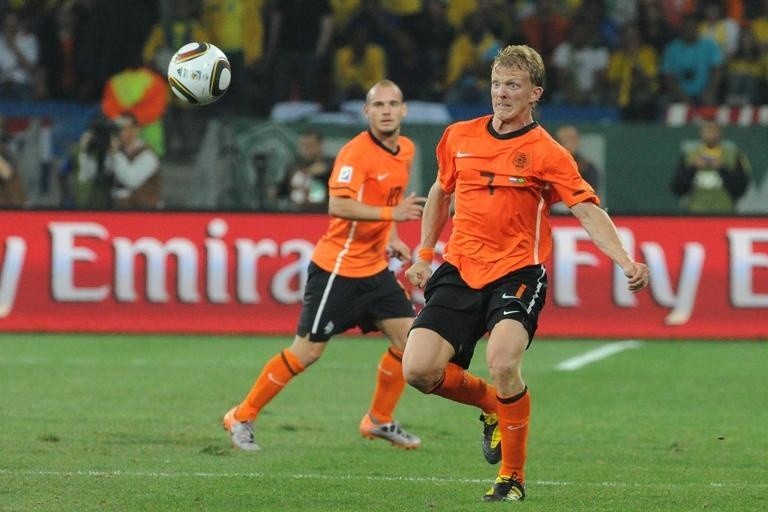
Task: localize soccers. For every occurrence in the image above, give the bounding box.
[167,42,233,105]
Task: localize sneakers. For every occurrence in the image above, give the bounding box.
[358,413,420,450]
[480,477,525,503]
[479,410,504,466]
[221,405,261,452]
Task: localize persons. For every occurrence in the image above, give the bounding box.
[0,1,767,211]
[222,77,428,452]
[399,43,649,499]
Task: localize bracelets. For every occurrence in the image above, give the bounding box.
[418,248,435,265]
